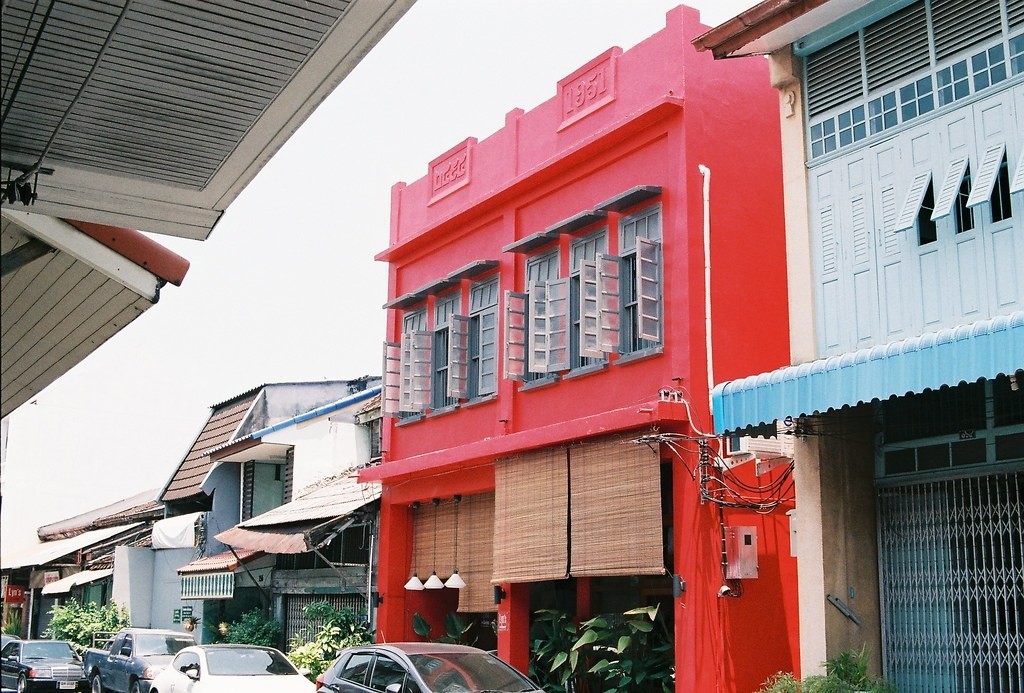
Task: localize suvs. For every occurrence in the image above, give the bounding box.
[315,642,546,693]
[148,643,317,693]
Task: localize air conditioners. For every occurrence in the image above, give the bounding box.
[726,417,796,458]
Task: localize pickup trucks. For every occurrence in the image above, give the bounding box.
[83,627,197,693]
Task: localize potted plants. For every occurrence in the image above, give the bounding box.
[185,616,201,631]
[219,622,228,635]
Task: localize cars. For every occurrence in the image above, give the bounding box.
[0,639,92,693]
[0,634,22,650]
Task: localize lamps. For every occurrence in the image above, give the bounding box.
[404,501,425,590]
[444,494,467,588]
[423,497,444,588]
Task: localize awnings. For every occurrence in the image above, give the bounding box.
[177,509,371,605]
[712,310,1024,437]
[41,570,113,595]
